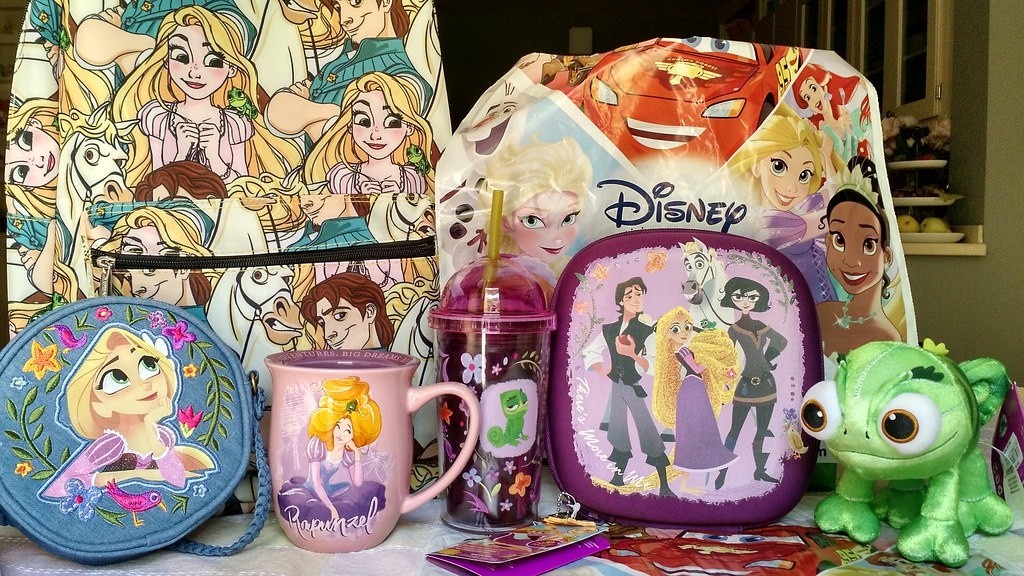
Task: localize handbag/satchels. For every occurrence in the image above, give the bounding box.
[0,296,274,565]
[443,40,919,493]
[545,228,825,525]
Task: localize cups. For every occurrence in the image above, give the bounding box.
[428,256,557,533]
[265,349,483,554]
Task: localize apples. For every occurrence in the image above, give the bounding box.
[898,215,950,233]
